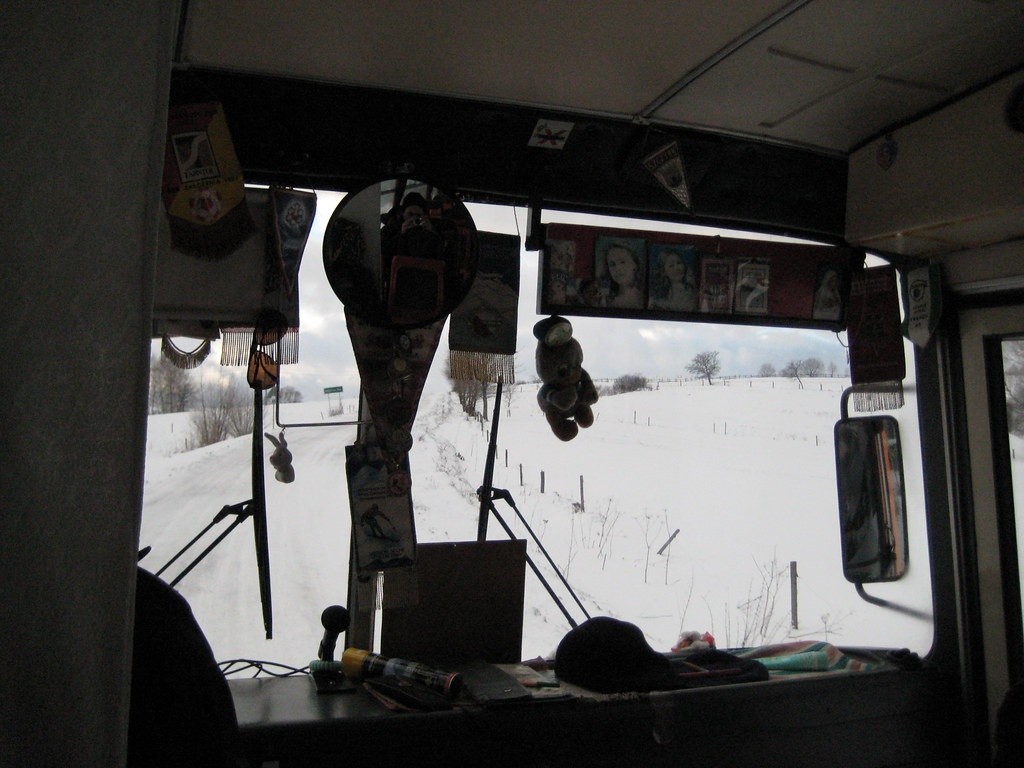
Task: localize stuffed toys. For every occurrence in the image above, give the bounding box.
[532,315,599,442]
[264,425,296,483]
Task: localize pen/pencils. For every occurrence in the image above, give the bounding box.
[520,680,560,689]
[309,660,341,671]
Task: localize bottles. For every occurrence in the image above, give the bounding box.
[340,648,461,694]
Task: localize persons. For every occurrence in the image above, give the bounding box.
[133,546,248,768]
[359,504,393,538]
[383,192,445,260]
[602,240,701,312]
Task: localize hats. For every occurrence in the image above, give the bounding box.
[553,617,676,694]
[402,192,426,212]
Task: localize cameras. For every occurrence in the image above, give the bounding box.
[413,216,427,226]
